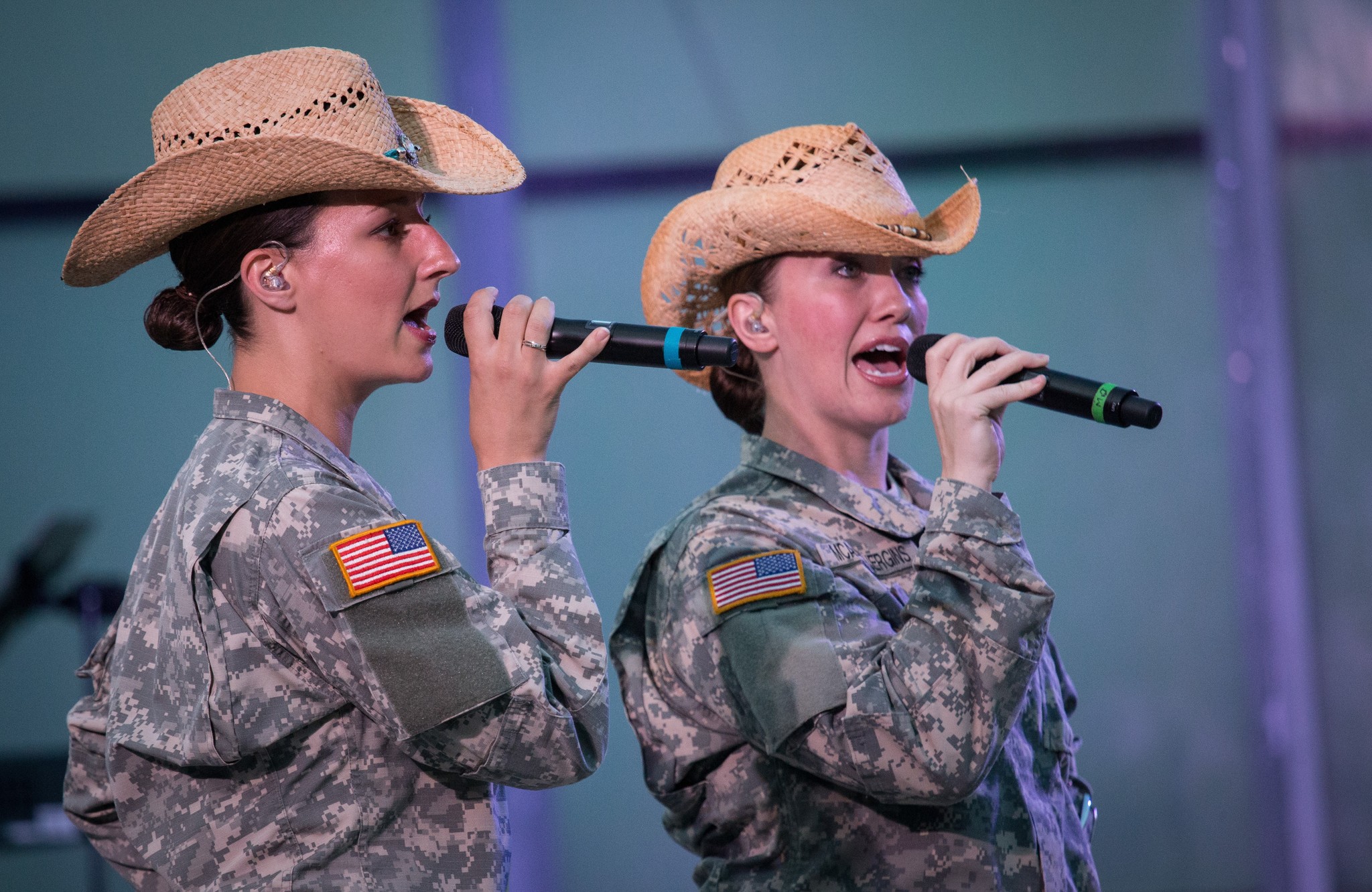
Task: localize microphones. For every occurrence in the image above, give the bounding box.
[442,304,741,370]
[907,333,1164,430]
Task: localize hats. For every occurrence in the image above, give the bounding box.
[639,122,985,394]
[62,53,523,288]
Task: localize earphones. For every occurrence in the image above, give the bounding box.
[261,264,285,290]
[746,309,767,333]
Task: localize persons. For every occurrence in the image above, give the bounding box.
[61,41,611,890]
[608,119,1105,889]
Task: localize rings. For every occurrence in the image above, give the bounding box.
[522,340,546,353]
